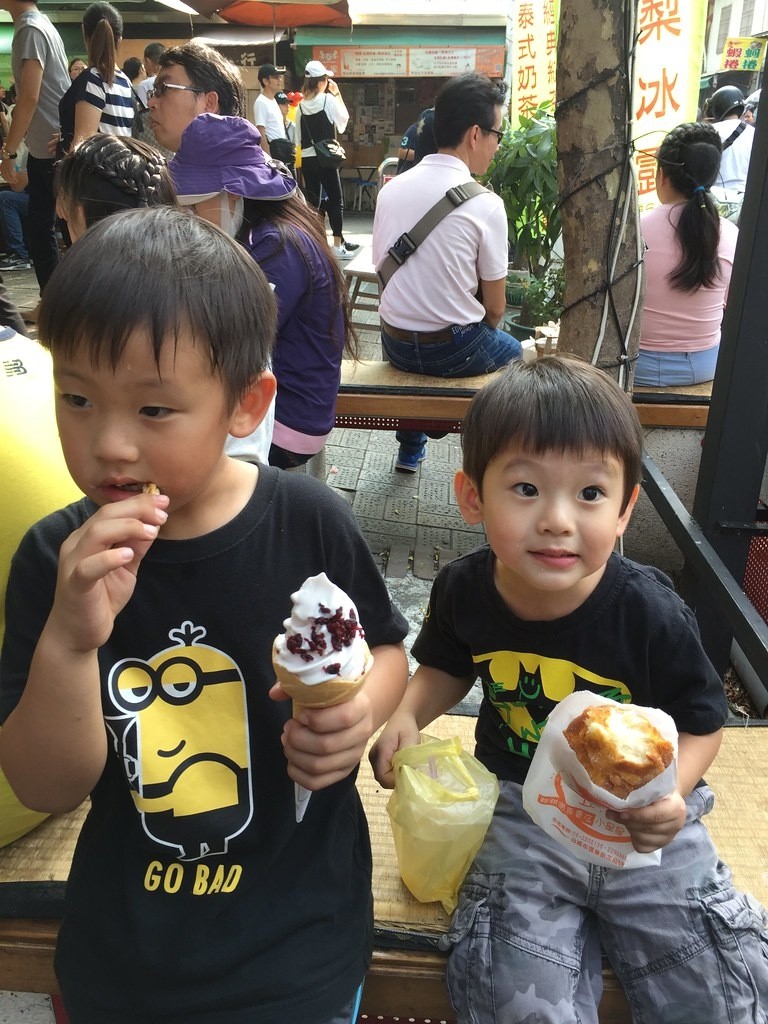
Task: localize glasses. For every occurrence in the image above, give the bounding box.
[472,122,504,145]
[264,76,281,81]
[145,84,210,100]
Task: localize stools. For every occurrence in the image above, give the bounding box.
[341,178,377,212]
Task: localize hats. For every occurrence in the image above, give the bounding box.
[258,65,287,81]
[305,60,334,79]
[165,112,297,205]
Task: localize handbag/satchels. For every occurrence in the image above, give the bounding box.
[314,138,346,170]
[386,733,500,916]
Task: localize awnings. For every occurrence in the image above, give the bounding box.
[292,24,507,80]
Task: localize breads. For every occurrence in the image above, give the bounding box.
[563,703,673,801]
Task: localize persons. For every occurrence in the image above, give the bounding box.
[56,135,177,241]
[373,73,523,472]
[0,208,410,1024]
[370,358,768,1024]
[123,57,147,86]
[633,123,738,386]
[137,43,166,109]
[58,2,135,169]
[147,44,247,151]
[297,60,363,259]
[696,86,761,191]
[274,92,296,148]
[167,113,357,469]
[253,64,295,180]
[68,58,88,80]
[0,0,73,337]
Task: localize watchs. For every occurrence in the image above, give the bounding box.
[0,150,18,160]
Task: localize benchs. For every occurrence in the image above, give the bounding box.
[284,360,712,572]
[342,246,382,331]
[0,728,768,1024]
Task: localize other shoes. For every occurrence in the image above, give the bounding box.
[344,242,358,251]
[18,299,43,322]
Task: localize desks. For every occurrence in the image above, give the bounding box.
[342,166,378,210]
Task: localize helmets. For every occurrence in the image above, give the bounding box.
[710,85,744,121]
[706,98,713,118]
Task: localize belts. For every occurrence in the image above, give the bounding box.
[379,317,455,345]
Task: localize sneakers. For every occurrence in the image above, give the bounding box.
[394,448,426,474]
[333,245,355,260]
[0,253,31,271]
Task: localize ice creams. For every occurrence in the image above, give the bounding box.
[271,572,374,721]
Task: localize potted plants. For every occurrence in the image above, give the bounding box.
[471,99,567,342]
